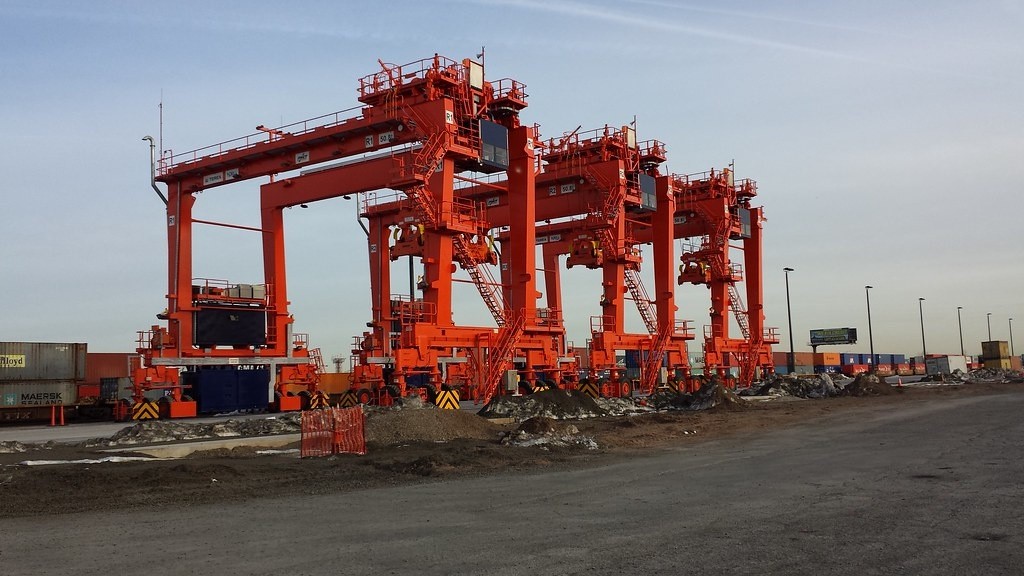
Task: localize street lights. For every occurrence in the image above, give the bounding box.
[957,306,965,356]
[864,285,876,371]
[919,297,929,376]
[783,266,796,372]
[1008,318,1014,356]
[987,313,993,341]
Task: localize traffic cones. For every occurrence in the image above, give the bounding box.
[46,404,58,427]
[57,404,69,427]
[897,378,902,387]
